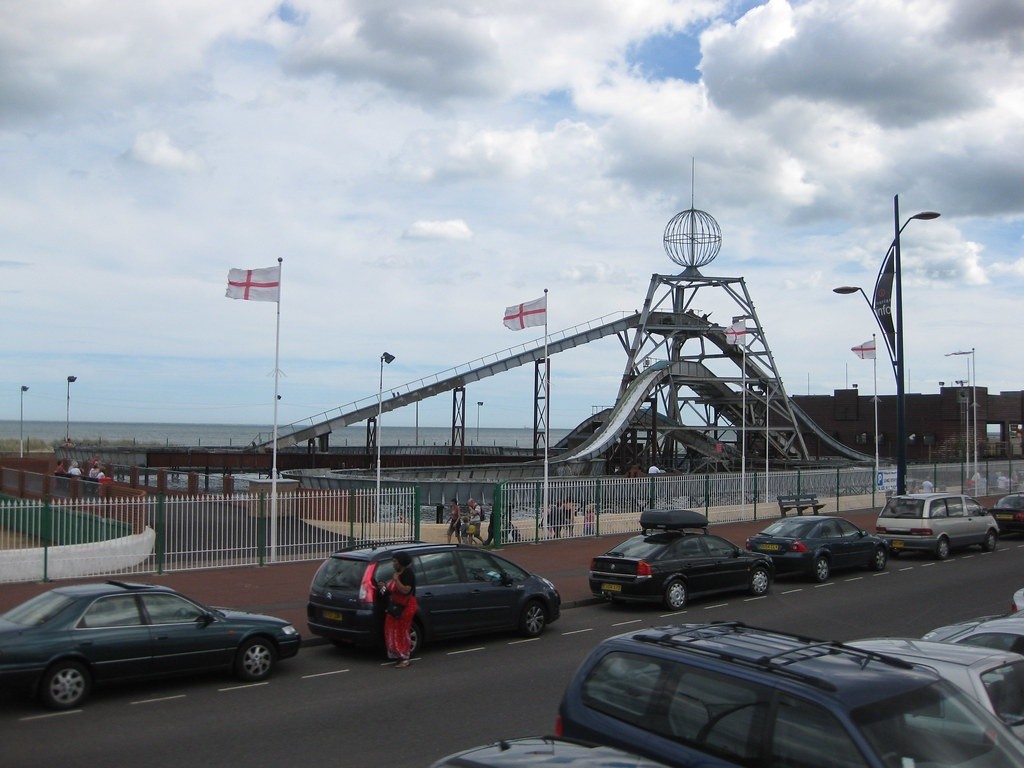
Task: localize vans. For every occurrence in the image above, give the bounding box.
[876,492,1000,561]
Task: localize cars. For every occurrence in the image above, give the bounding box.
[986,494,1024,532]
[427,734,669,768]
[919,612,1024,659]
[842,636,1024,747]
[1011,586,1024,613]
[586,510,776,610]
[0,579,301,712]
[745,515,890,583]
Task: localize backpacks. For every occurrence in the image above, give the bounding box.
[471,505,485,521]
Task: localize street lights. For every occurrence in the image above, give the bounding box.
[477,401,483,441]
[375,351,397,525]
[833,193,942,496]
[19,386,30,458]
[66,376,78,442]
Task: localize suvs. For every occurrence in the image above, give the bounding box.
[307,540,562,656]
[555,621,1024,768]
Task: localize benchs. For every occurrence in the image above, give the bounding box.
[777,493,824,518]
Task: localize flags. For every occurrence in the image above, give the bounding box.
[723,321,745,345]
[224,266,281,302]
[851,340,876,359]
[504,294,547,331]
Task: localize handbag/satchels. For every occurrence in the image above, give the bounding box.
[388,601,405,619]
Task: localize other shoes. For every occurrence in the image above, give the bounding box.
[395,660,410,668]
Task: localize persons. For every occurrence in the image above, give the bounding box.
[57,456,105,478]
[884,469,1018,502]
[446,498,521,545]
[377,551,417,667]
[540,498,595,539]
